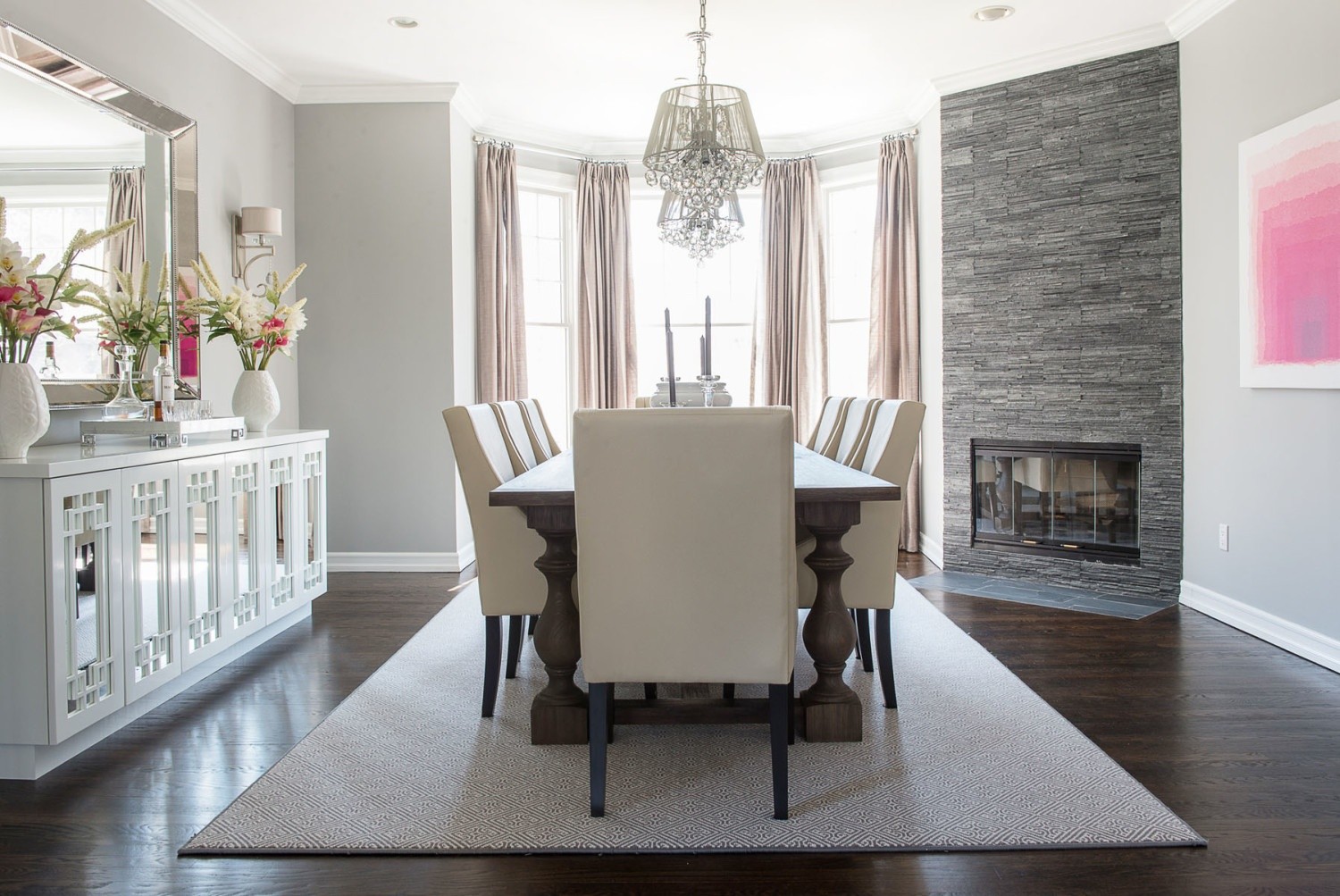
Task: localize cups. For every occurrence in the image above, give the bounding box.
[161,400,210,421]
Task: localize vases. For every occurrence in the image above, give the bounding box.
[232,370,280,432]
[0,363,50,459]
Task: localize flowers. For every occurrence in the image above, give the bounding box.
[66,250,171,372]
[179,253,308,370]
[0,201,138,363]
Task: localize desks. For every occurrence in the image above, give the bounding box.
[489,443,901,743]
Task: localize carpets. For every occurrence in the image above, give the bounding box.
[178,573,1210,857]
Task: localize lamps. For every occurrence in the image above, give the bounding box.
[643,0,765,266]
[231,206,282,296]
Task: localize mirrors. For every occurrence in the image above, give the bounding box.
[0,59,177,379]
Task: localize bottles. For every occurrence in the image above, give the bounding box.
[102,345,149,421]
[41,341,60,379]
[152,340,176,421]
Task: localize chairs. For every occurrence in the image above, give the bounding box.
[444,396,926,821]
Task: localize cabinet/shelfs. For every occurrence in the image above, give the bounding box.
[0,430,329,779]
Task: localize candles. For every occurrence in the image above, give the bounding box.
[664,307,676,407]
[700,295,711,375]
